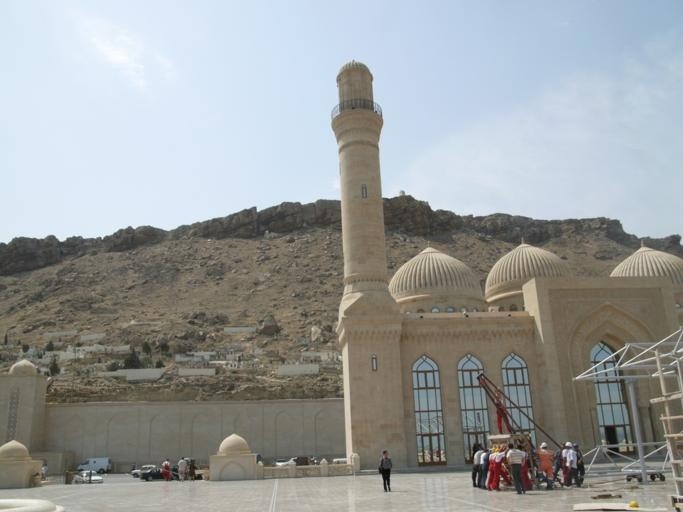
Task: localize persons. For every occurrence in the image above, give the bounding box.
[40,463,45,481]
[161,456,170,481]
[177,456,187,480]
[377,449,392,493]
[189,458,199,480]
[470,440,585,493]
[42,463,48,475]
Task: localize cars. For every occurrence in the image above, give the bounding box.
[130,464,155,477]
[74,470,102,482]
[138,466,178,481]
[271,458,287,467]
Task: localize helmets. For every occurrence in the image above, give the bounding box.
[564,442,572,447]
[541,442,548,447]
[573,443,579,449]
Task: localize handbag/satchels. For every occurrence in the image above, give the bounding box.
[378,466,384,474]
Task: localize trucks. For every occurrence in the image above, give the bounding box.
[76,457,111,473]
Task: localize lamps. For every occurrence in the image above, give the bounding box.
[371,353,377,372]
